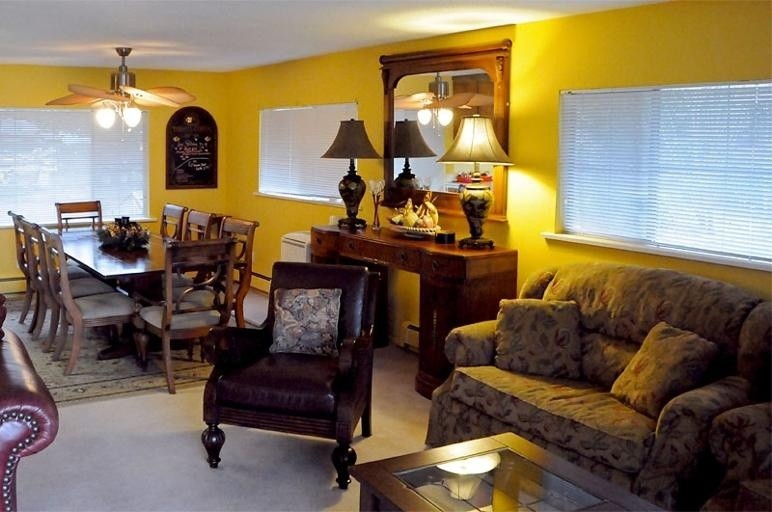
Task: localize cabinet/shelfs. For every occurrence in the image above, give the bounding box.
[310,229,517,400]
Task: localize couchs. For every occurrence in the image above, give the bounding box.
[0,293,58,512]
[425,263,772,510]
[699,402,772,511]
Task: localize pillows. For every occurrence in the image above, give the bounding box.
[269,287,343,358]
[611,321,718,421]
[494,297,583,382]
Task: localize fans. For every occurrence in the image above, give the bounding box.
[44,47,197,110]
[394,72,494,109]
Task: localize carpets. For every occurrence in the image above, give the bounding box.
[3,311,265,407]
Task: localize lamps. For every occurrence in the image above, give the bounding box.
[435,451,501,502]
[432,114,515,253]
[320,118,382,230]
[392,118,436,189]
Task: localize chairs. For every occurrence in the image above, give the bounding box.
[39,228,142,376]
[18,216,118,352]
[7,211,65,333]
[134,215,259,359]
[160,202,190,241]
[202,261,382,489]
[55,200,103,234]
[138,237,237,395]
[139,209,216,298]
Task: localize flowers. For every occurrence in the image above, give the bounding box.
[97,219,149,255]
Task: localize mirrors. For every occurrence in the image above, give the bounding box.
[379,39,512,223]
